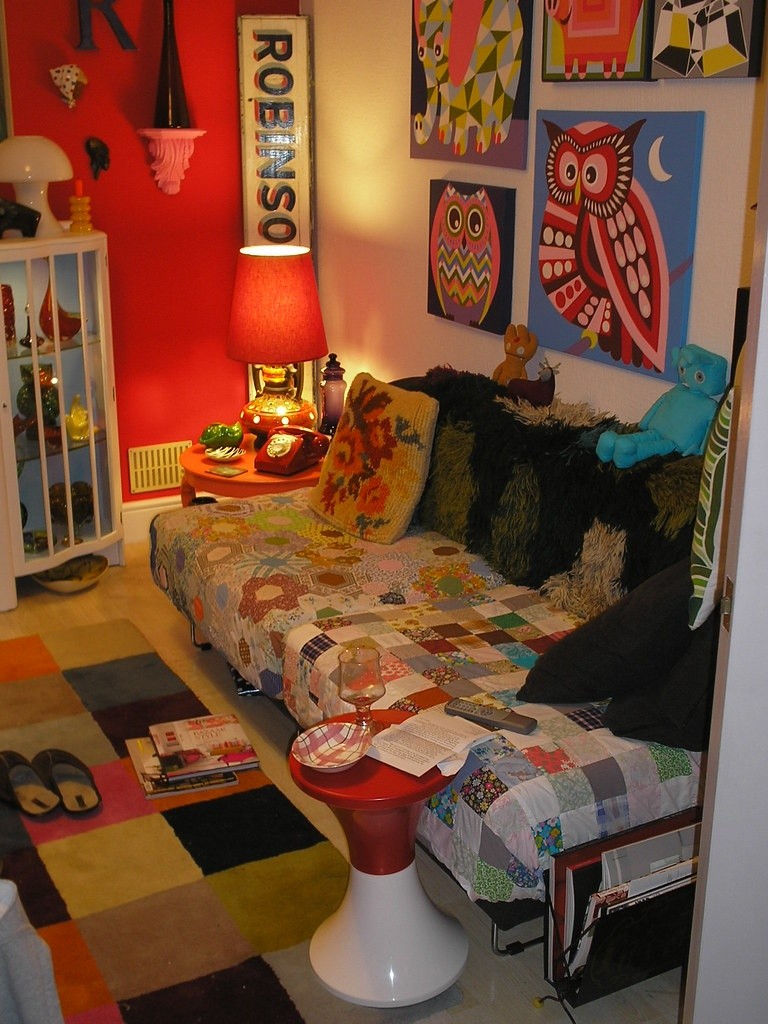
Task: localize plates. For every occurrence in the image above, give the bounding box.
[205,447,246,461]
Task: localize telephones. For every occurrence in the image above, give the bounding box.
[254,424,329,476]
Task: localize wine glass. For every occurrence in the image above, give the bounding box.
[338,647,386,736]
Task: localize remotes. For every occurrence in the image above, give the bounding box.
[444,697,537,735]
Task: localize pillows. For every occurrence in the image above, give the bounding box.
[310,372,440,546]
[600,627,720,750]
[518,553,715,704]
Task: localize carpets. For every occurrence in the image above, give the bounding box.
[0,619,353,1024]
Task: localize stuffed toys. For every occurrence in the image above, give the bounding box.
[493,324,537,387]
[597,344,727,469]
[506,358,561,407]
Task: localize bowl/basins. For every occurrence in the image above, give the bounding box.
[32,554,109,593]
[23,531,57,555]
[292,722,371,773]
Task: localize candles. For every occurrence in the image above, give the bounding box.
[75,178,84,198]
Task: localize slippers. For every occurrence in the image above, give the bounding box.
[0,750,60,816]
[31,749,101,813]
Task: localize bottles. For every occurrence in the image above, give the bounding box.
[319,353,347,437]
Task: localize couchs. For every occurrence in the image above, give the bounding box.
[151,368,727,956]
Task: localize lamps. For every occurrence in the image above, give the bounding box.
[0,134,73,236]
[222,245,329,447]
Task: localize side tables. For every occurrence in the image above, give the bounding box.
[291,709,471,1008]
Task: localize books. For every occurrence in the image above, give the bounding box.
[148,713,260,782]
[125,736,239,799]
